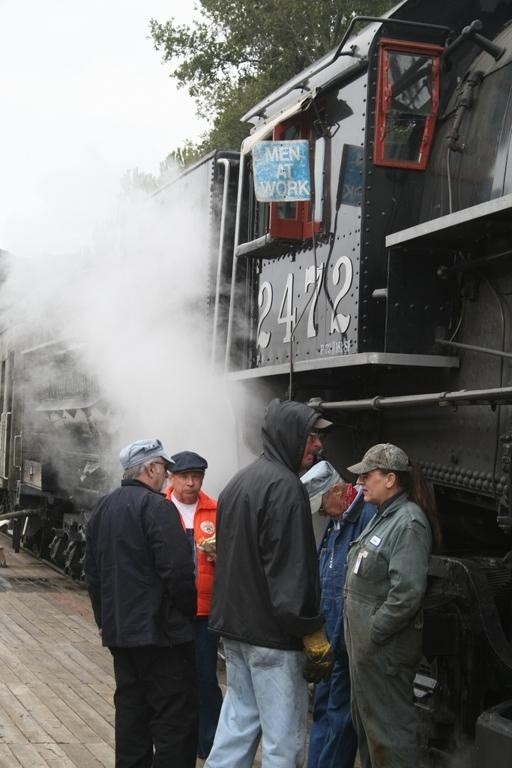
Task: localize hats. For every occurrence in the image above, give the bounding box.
[165,450,209,475]
[300,459,343,515]
[346,441,415,475]
[119,438,176,469]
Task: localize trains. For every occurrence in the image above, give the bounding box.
[0,0,512,768]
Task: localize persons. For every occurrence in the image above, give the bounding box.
[83,439,199,765]
[199,399,335,765]
[300,459,380,768]
[162,452,225,765]
[341,445,433,767]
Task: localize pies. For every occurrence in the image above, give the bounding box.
[200,533,216,550]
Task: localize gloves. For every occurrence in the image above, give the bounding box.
[301,628,334,666]
